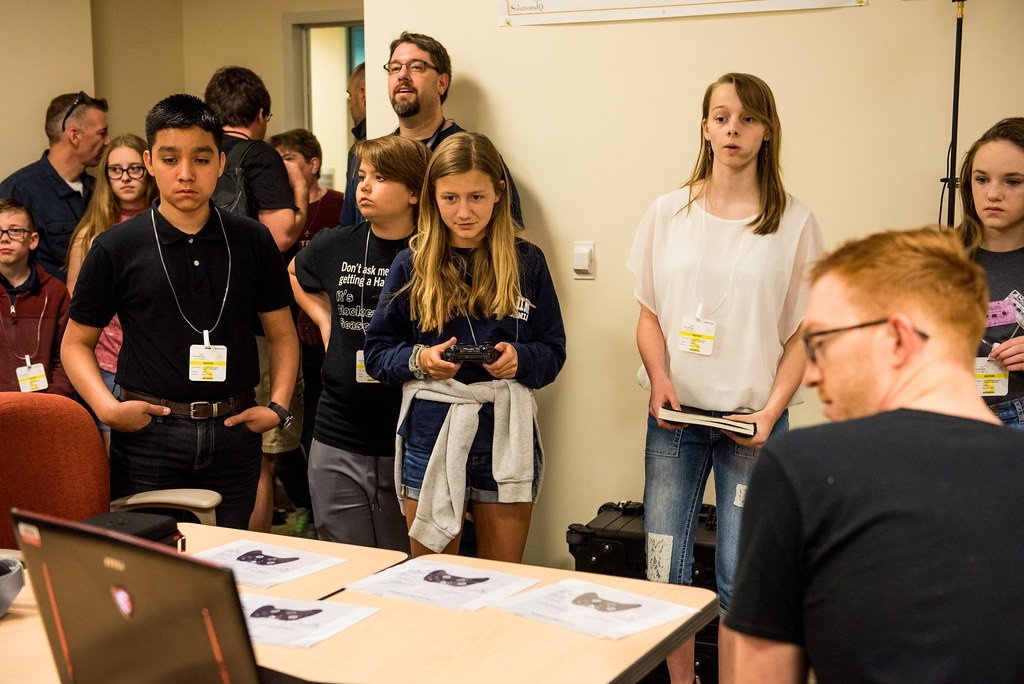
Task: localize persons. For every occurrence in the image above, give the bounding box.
[0,33,524,559]
[634,73,820,684]
[362,130,568,569]
[731,225,1023,684]
[954,117,1024,433]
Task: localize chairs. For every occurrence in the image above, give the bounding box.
[0,391,222,551]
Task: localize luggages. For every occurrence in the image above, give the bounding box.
[567,500,719,684]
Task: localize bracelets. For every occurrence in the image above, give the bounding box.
[416,344,430,376]
[408,343,427,380]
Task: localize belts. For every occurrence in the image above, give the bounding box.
[119,386,256,419]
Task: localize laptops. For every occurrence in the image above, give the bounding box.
[7,508,325,684]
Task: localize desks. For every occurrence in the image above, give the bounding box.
[0,523,720,684]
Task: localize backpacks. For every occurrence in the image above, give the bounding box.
[212,139,268,215]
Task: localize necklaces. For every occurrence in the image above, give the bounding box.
[303,187,321,238]
[223,130,252,142]
[446,247,473,278]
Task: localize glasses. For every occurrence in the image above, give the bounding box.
[266,113,273,122]
[0,229,32,240]
[106,166,146,180]
[62,91,91,132]
[802,317,930,363]
[383,59,438,74]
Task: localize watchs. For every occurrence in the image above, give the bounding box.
[266,401,295,432]
